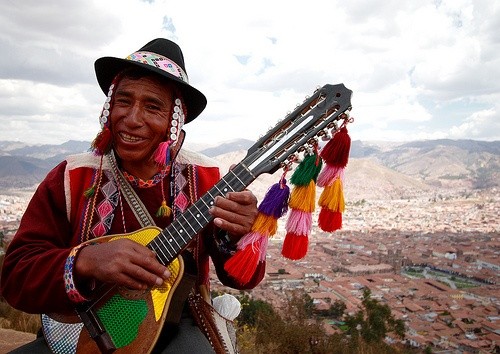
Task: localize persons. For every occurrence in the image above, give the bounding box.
[0,37,266,354]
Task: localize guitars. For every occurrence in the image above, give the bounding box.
[42,81,352,354]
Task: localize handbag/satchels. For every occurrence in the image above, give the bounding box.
[190,293,238,354]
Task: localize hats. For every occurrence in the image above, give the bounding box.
[95,38,207,124]
